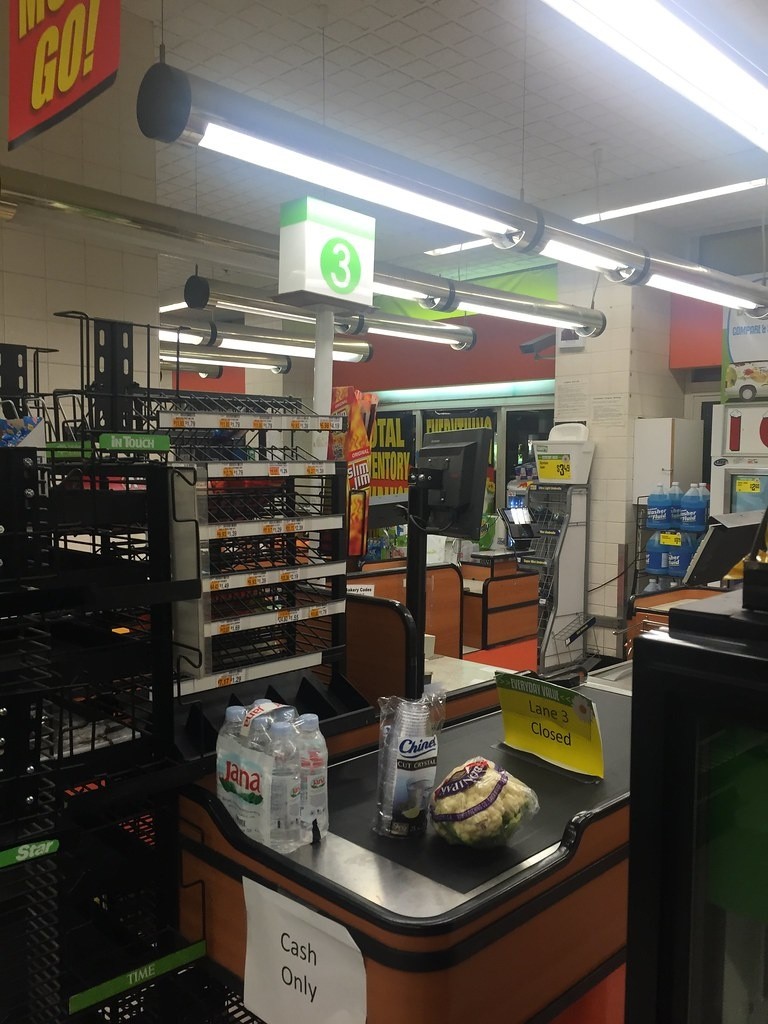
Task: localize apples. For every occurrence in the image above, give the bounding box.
[725,367,737,387]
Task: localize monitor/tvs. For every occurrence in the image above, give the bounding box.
[500,506,537,525]
[416,427,493,542]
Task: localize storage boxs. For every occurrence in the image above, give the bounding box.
[532,439,596,484]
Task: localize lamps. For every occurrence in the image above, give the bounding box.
[134,0,768,380]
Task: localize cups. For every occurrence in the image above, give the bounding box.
[380,702,430,839]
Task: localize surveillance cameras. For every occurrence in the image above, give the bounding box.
[520,333,556,354]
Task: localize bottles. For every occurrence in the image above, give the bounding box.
[644,482,710,593]
[216,699,329,855]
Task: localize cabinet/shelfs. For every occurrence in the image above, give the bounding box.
[631,496,709,595]
[0,331,352,1024]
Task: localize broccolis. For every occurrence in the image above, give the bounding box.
[428,755,533,848]
